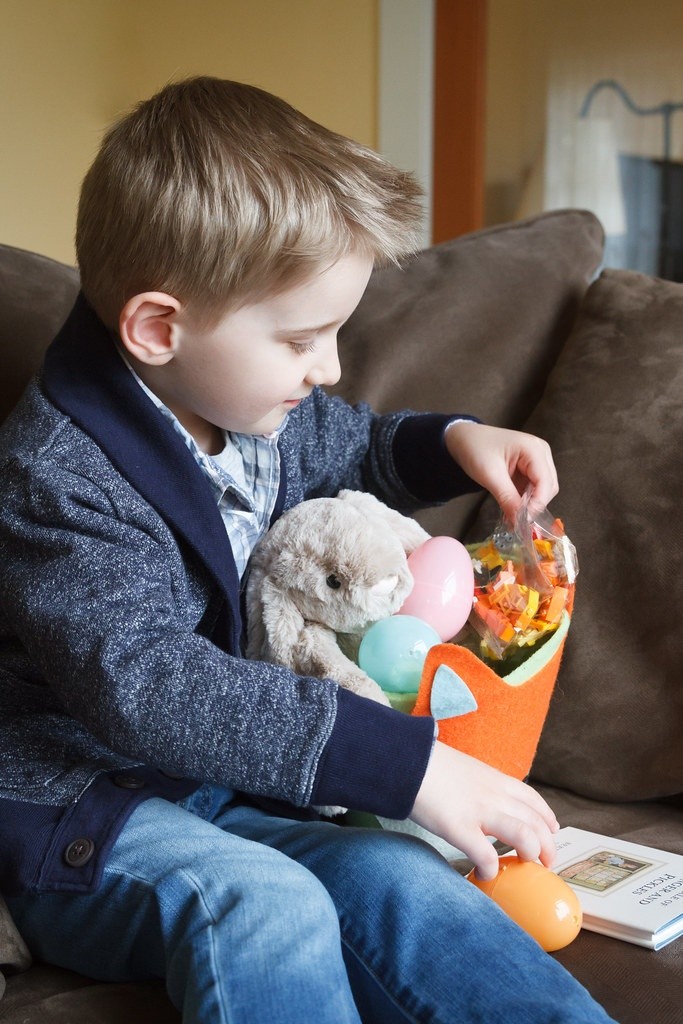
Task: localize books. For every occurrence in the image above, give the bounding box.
[463,826,683,952]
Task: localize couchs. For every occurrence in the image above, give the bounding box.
[0,768,683,1024]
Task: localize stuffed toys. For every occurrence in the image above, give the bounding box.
[239,489,433,818]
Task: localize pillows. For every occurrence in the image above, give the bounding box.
[0,206,608,539]
[471,266,683,802]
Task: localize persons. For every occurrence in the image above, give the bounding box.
[1,75,621,1024]
